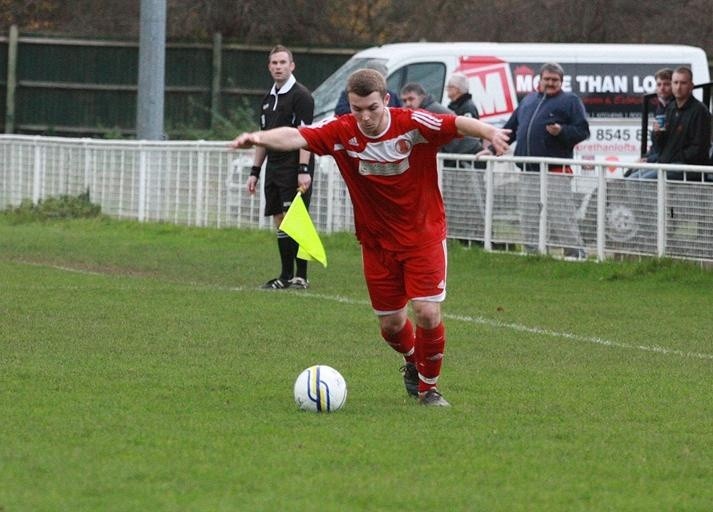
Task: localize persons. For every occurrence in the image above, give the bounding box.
[245,43,315,289]
[229,69,514,410]
[335,60,401,116]
[401,81,506,251]
[476,63,592,262]
[623,68,673,176]
[625,66,713,239]
[448,75,485,248]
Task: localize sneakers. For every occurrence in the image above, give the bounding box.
[398,361,449,406]
[260,277,310,289]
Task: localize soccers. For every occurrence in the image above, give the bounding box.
[293,365,348,412]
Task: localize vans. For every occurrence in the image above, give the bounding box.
[226,40,713,250]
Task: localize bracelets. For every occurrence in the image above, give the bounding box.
[298,163,310,174]
[251,166,261,178]
